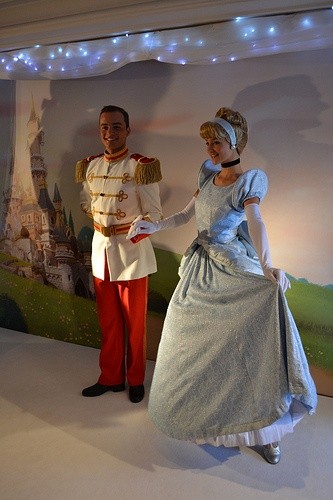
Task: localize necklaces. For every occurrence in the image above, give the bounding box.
[221,158,240,168]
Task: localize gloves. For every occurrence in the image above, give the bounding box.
[244,203,291,293]
[125,215,142,239]
[127,196,196,235]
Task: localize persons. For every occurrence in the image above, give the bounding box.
[74,106,163,404]
[125,107,317,465]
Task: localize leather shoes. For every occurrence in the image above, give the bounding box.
[81,382,125,397]
[129,383,144,403]
[263,443,280,464]
